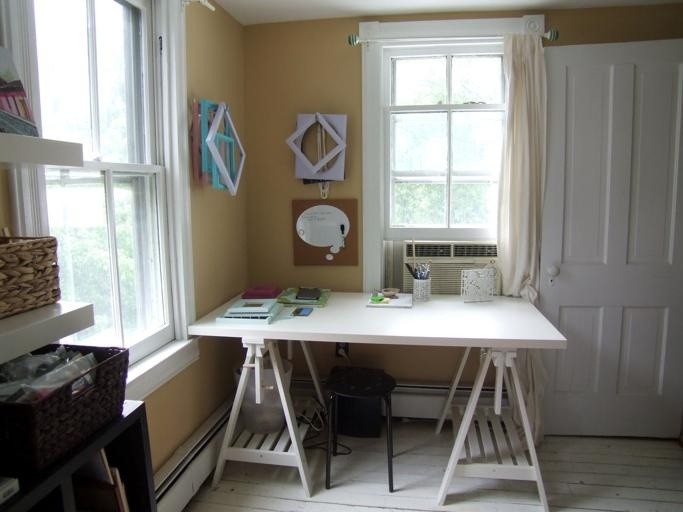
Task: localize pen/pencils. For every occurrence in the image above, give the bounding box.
[404,260,431,280]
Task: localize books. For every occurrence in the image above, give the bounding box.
[277,285,332,309]
[366,288,414,309]
[295,286,322,301]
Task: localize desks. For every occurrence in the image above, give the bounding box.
[187,285,568,511]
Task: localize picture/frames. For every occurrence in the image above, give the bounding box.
[283,111,347,181]
[189,97,246,197]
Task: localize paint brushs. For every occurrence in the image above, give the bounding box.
[412,236,415,272]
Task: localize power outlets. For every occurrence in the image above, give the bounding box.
[334,343,350,360]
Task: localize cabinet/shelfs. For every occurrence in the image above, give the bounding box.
[1,131,157,511]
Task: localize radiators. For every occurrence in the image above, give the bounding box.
[403,241,502,294]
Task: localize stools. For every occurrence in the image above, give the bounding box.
[324,365,398,493]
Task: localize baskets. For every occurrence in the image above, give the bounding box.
[0,235,61,319]
[0,343,130,476]
